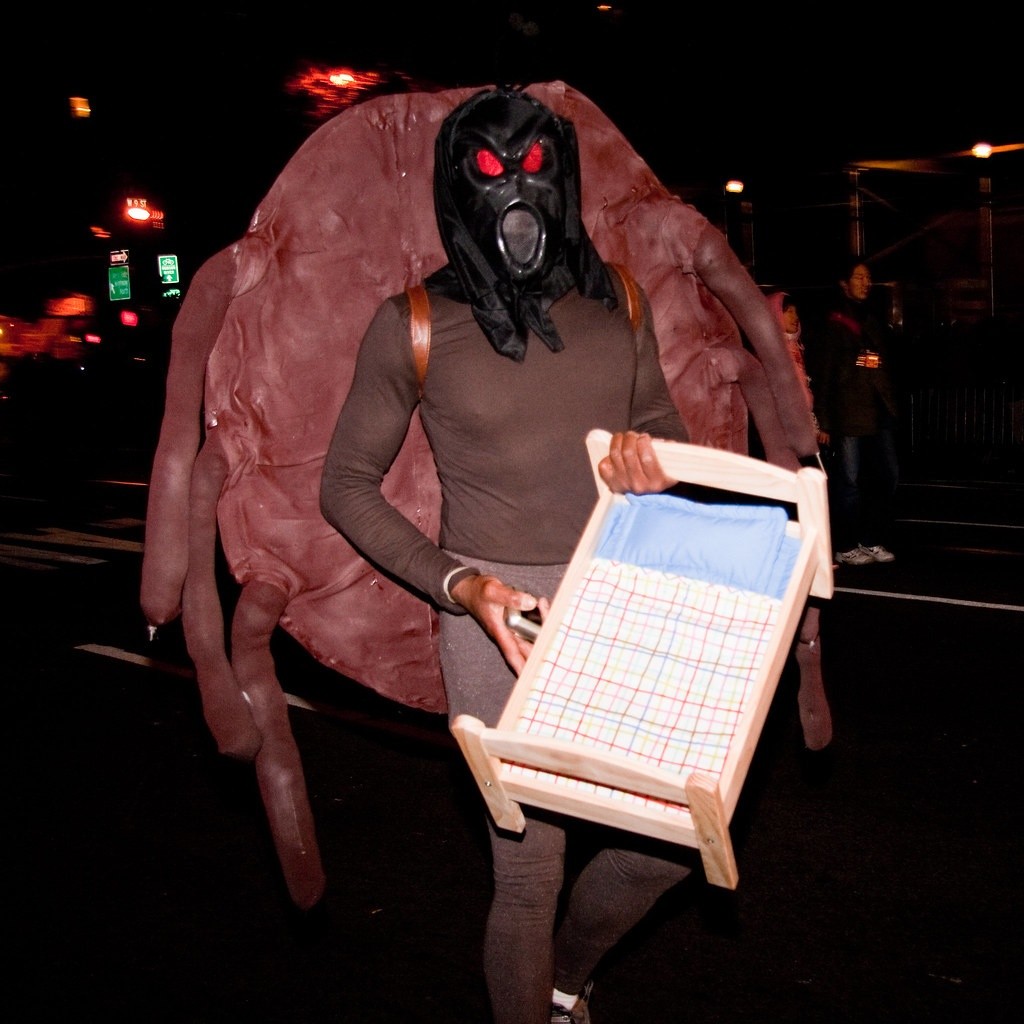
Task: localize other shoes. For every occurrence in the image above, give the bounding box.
[549,980,594,1024]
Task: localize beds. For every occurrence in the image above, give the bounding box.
[449,428,834,890]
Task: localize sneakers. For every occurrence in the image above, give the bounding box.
[857,542,895,562]
[835,548,876,564]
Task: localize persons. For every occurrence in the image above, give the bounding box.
[766,256,907,575]
[321,91,702,1024]
